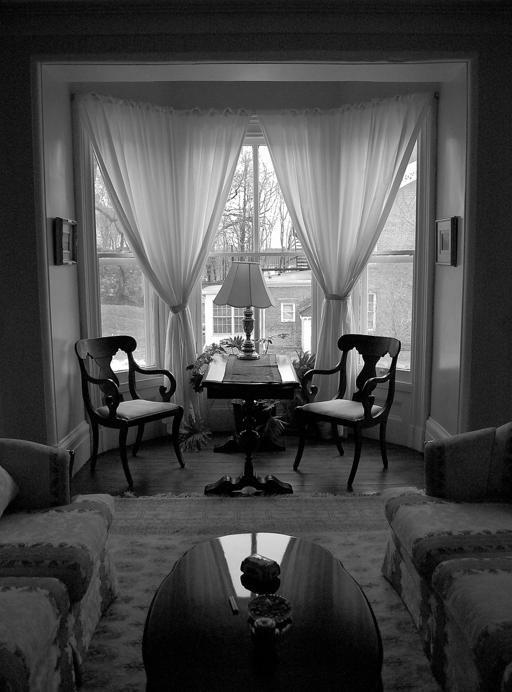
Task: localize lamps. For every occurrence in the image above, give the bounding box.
[212,261,275,361]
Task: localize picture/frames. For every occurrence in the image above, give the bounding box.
[55,216,79,266]
[435,216,458,267]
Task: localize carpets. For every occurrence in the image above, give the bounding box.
[79,486,442,691]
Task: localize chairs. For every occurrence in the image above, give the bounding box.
[293,333,401,492]
[75,335,185,494]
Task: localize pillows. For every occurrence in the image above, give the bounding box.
[0,466,21,519]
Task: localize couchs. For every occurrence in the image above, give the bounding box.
[380,419,512,691]
[0,437,117,691]
